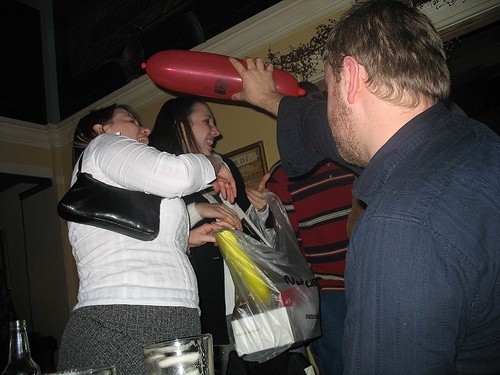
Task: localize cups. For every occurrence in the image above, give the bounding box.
[45,364,117,375]
[143,333,214,375]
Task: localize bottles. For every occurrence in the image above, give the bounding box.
[1,319,41,375]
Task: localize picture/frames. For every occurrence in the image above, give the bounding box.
[223,140,269,192]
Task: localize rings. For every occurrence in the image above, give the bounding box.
[209,224,213,229]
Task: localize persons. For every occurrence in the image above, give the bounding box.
[147,95,314,375]
[228,3,500,375]
[57,104,236,375]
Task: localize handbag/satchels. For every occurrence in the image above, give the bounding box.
[211,190,323,364]
[56,149,163,242]
